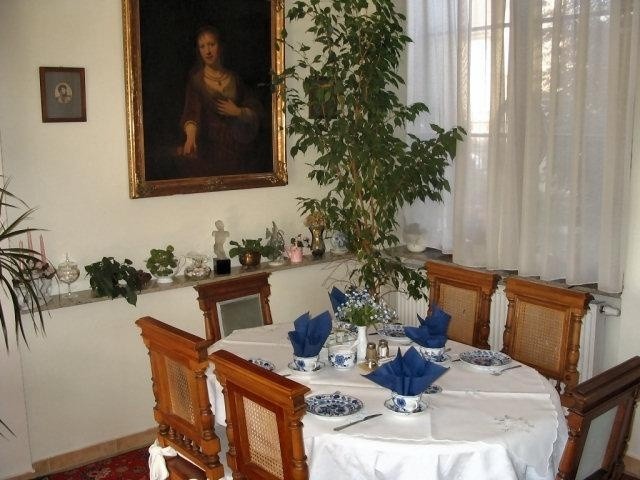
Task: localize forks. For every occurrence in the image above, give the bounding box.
[493,363,523,375]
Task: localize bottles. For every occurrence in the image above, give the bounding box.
[308,225,326,257]
[184,255,212,281]
[366,339,390,364]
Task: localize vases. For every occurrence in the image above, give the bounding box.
[357,326,368,363]
[306,224,329,260]
[290,246,304,263]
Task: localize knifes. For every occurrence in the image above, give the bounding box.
[333,412,383,432]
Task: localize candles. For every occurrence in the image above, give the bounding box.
[19,227,46,271]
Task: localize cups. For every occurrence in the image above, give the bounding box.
[293,354,319,369]
[391,391,422,411]
[329,345,357,372]
[419,345,443,361]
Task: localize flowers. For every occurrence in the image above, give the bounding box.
[335,288,398,327]
[286,234,310,248]
[304,211,330,229]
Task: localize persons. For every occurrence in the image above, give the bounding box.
[180,26,259,176]
[57,85,72,104]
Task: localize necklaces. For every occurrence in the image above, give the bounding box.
[203,68,228,85]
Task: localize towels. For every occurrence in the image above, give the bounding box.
[287,309,332,357]
[404,303,452,348]
[328,286,348,314]
[360,346,450,396]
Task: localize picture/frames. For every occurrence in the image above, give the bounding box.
[121,0,288,200]
[39,67,87,123]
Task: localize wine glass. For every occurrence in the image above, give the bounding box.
[58,261,82,299]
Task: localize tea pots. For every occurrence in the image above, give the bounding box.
[285,241,303,263]
[143,256,186,284]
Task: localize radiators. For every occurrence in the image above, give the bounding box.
[397,268,606,393]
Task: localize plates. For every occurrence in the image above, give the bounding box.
[424,354,451,363]
[422,384,444,394]
[287,362,326,373]
[304,392,363,418]
[247,358,276,372]
[383,397,428,415]
[460,350,511,368]
[378,325,417,343]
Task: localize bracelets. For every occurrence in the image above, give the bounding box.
[240,107,244,119]
[184,121,200,131]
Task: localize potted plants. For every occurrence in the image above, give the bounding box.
[146,245,186,284]
[228,237,276,267]
[84,256,151,306]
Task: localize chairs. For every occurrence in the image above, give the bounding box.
[501,276,595,391]
[193,271,273,341]
[210,349,311,480]
[554,356,640,479]
[424,259,502,350]
[135,316,226,480]
